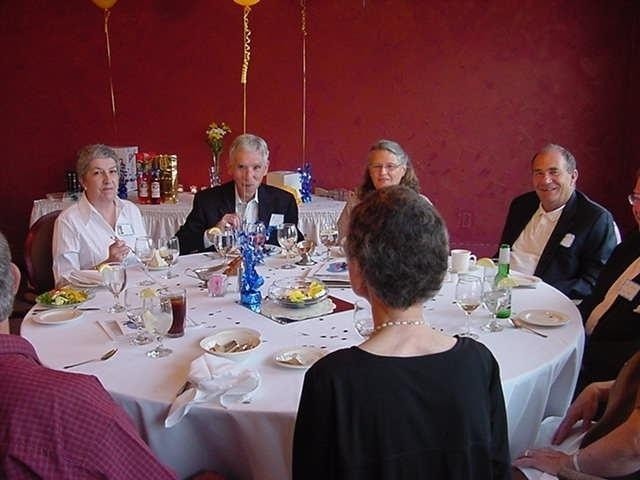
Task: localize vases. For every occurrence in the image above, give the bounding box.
[207,166,222,189]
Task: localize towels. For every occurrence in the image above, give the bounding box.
[519,416,599,480]
[165,354,261,428]
[70,270,105,287]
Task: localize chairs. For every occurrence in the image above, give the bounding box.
[24,210,64,295]
[315,186,356,202]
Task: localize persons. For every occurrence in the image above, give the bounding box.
[0,231,181,480]
[511,354,640,480]
[493,144,617,306]
[570,181,640,408]
[167,134,305,256]
[292,185,511,480]
[53,144,152,289]
[337,140,449,253]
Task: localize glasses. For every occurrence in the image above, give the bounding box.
[369,161,403,171]
[628,193,639,204]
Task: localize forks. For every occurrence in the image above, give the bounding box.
[63,348,118,370]
[509,318,548,338]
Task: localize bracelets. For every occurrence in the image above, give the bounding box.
[573,453,581,473]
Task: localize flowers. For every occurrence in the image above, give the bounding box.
[205,120,232,175]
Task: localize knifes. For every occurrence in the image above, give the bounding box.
[33,307,101,312]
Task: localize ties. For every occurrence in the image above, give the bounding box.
[586,256,640,335]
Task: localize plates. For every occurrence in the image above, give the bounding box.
[31,308,84,325]
[199,327,263,357]
[511,273,542,286]
[272,346,327,370]
[71,280,100,288]
[34,288,95,308]
[518,309,570,327]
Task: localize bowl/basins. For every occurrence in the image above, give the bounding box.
[265,276,330,308]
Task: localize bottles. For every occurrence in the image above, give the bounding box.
[492,244,512,319]
[148,159,160,204]
[137,162,149,204]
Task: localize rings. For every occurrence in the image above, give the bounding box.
[525,449,529,457]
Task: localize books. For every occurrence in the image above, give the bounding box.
[304,257,352,288]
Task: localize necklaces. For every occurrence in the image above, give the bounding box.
[373,321,429,333]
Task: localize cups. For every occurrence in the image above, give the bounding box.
[214,231,236,265]
[124,287,154,346]
[144,298,174,359]
[454,273,483,341]
[319,222,339,262]
[276,223,298,270]
[135,236,157,286]
[102,262,127,314]
[166,287,187,338]
[450,249,477,274]
[480,276,512,333]
[247,222,267,252]
[298,240,316,265]
[352,299,374,341]
[159,236,180,279]
